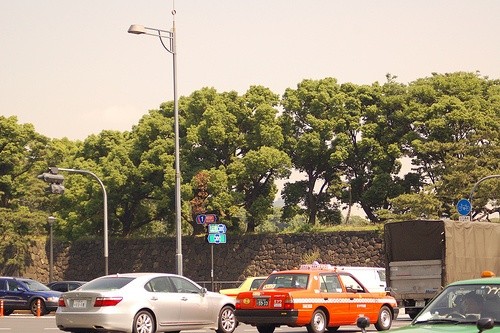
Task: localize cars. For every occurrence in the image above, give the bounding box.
[233,261,403,330]
[218,273,304,299]
[55,273,240,332]
[0,276,68,315]
[46,281,90,293]
[381,271,500,333]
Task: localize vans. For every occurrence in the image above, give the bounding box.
[333,266,388,293]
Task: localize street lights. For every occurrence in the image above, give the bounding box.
[49,215,56,283]
[128,24,183,276]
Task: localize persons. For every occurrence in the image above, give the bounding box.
[460,291,496,321]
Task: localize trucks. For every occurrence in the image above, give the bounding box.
[383,219,500,320]
